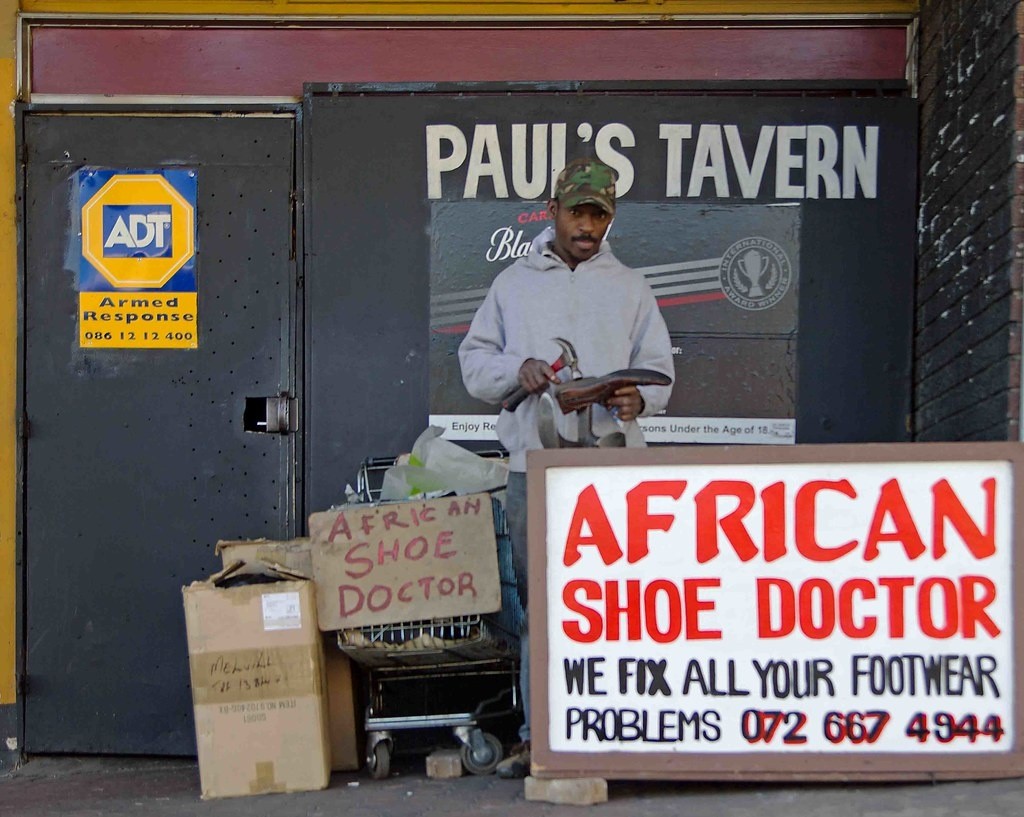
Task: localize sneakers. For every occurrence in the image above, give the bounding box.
[496,740,531,778]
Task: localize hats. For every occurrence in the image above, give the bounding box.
[554,159,616,216]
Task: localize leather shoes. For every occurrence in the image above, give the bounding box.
[554,368,672,414]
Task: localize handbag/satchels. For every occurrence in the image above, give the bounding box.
[379,424,509,499]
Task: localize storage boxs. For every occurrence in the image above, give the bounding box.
[221,539,359,771]
[182,561,331,800]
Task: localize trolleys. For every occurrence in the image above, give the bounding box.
[332,446,528,778]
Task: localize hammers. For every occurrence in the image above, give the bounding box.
[500,337,584,413]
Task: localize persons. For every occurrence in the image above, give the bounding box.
[457,158,676,779]
